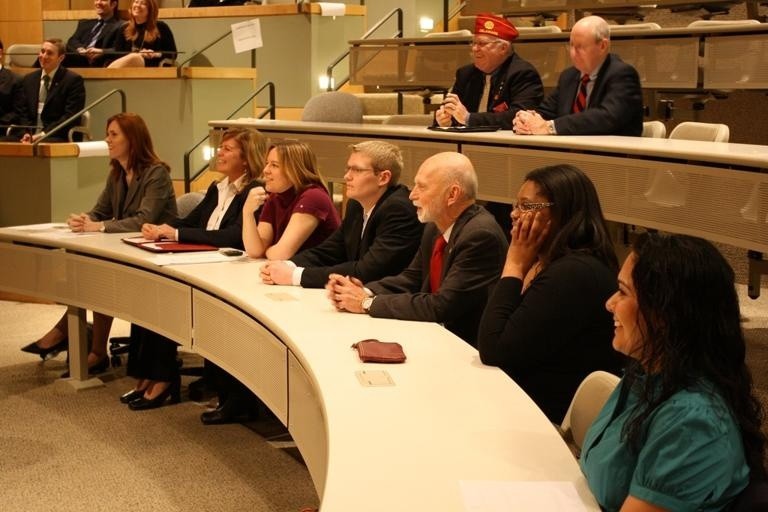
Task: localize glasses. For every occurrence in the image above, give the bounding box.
[344,165,373,174]
[512,201,555,211]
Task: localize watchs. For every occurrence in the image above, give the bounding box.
[360,295,376,314]
[548,121,555,135]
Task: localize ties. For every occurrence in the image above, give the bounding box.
[39,75,50,104]
[429,236,448,294]
[573,74,590,114]
[478,75,491,114]
[91,18,104,39]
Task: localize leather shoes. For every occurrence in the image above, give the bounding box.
[61,356,110,378]
[21,337,68,353]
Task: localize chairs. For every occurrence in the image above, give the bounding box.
[0,0,768,512]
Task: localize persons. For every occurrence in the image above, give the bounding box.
[201,136,339,422]
[20,112,178,376]
[118,128,270,410]
[572,230,757,512]
[326,150,509,349]
[478,162,624,408]
[263,138,422,293]
[1,0,179,141]
[512,15,645,134]
[430,11,545,129]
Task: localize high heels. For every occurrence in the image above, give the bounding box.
[120,376,181,410]
[201,397,259,424]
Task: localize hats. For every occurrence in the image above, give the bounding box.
[475,13,519,42]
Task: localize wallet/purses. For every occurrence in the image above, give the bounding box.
[352,338,406,364]
